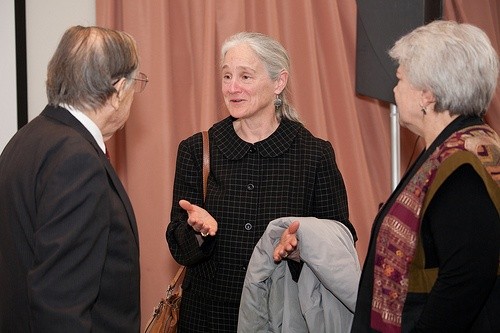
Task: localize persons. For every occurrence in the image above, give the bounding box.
[351,19,500,333]
[0,26,149,333]
[166,33,358,333]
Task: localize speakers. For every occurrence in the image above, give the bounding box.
[355,0,444,104]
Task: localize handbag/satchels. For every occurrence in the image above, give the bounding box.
[144,288,182,333]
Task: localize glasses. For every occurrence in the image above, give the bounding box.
[112,71,149,94]
